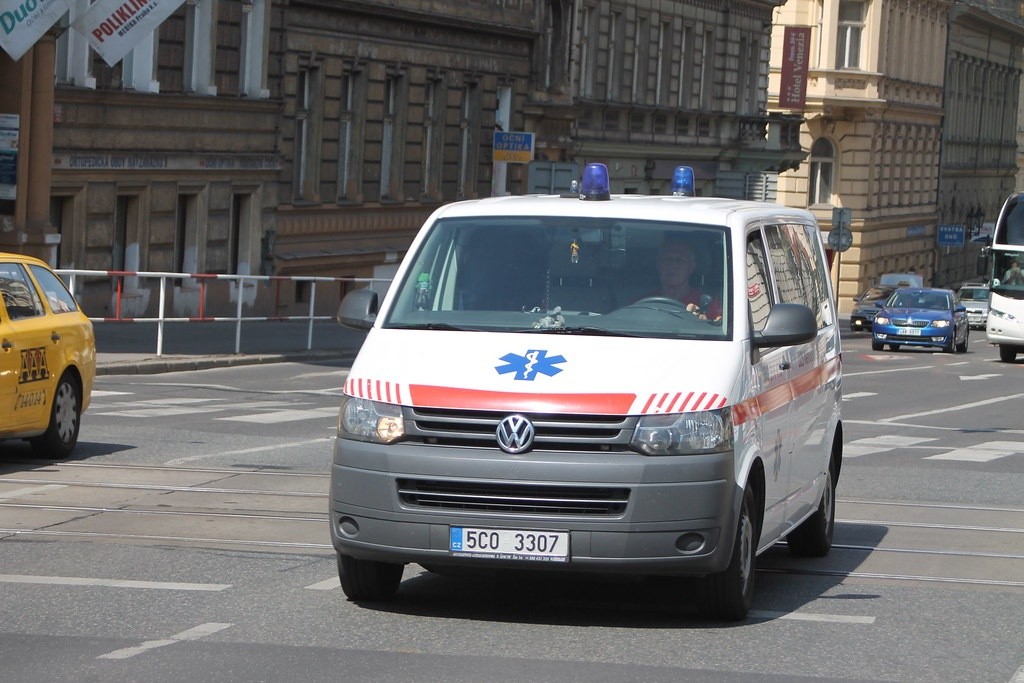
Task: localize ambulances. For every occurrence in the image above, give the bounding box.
[329,195,844,623]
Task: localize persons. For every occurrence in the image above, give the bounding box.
[902,294,914,308]
[929,296,946,310]
[1001,261,1024,285]
[630,236,723,325]
[519,268,590,312]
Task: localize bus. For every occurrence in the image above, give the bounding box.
[980,191,1024,363]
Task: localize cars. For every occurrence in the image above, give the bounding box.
[872,286,969,354]
[956,283,990,331]
[0,252,96,460]
[850,285,898,332]
